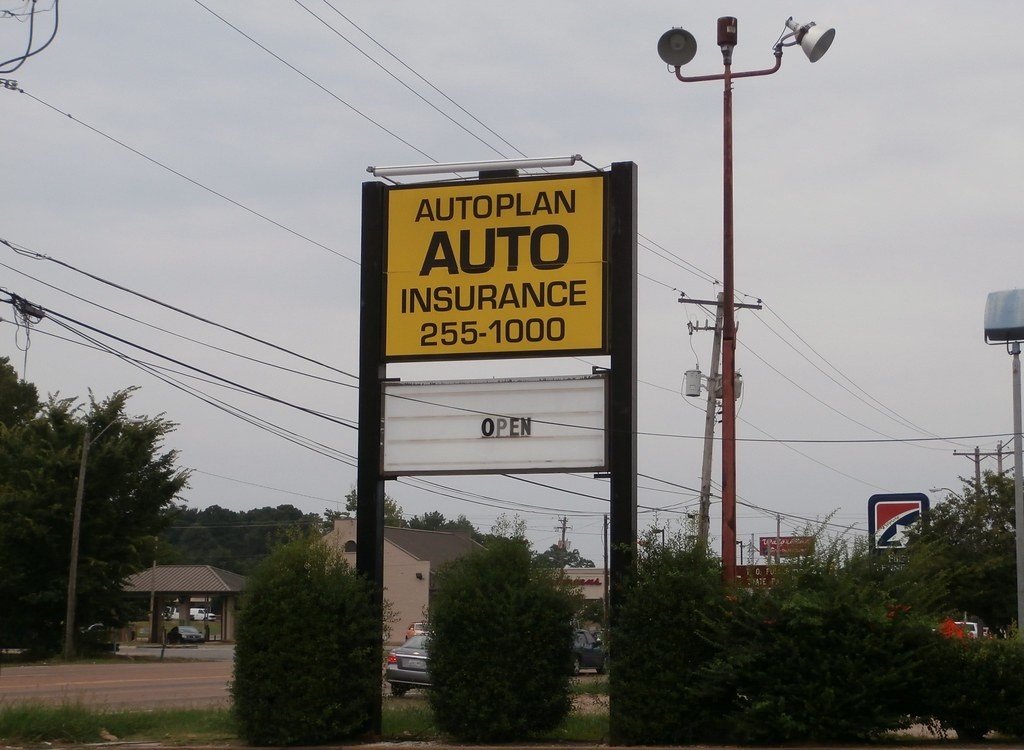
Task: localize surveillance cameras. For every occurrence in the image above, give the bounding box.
[685,369,702,397]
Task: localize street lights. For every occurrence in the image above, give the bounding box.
[64,417,145,656]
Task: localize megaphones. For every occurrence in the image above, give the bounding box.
[657,26,697,66]
[784,16,836,62]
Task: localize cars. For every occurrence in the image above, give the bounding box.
[166,626,204,642]
[571,628,606,675]
[189,607,216,622]
[384,633,434,696]
[405,620,428,642]
